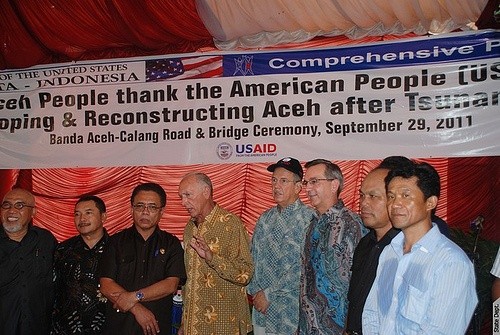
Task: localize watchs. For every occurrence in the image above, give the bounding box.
[135,289,144,302]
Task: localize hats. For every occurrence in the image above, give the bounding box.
[266,157,303,180]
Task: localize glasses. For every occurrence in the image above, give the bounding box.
[133,204,163,212]
[302,178,335,187]
[0,201,34,210]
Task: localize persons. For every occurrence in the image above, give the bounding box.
[51,195,111,335]
[361,161,478,335]
[0,189,59,335]
[246,156,315,335]
[178,171,254,335]
[299,159,369,335]
[347,156,479,335]
[98,180,187,335]
